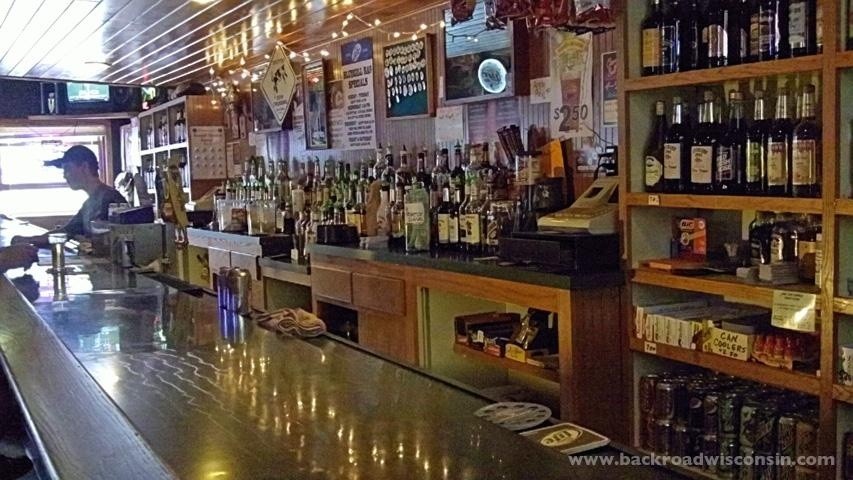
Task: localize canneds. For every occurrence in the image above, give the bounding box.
[638,367,817,480]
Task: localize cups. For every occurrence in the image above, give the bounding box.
[48,233,67,271]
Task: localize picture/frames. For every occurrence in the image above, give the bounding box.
[381,35,434,120]
[301,58,331,150]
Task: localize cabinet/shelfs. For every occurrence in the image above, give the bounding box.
[616,0,852,480]
[136,96,227,215]
[308,243,623,440]
[177,226,309,314]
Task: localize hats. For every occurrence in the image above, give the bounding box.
[43,145,98,168]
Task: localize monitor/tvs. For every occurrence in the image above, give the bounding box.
[64,80,110,103]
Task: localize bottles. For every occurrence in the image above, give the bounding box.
[644,99,669,193]
[717,0,730,66]
[493,141,507,202]
[428,184,441,259]
[481,179,489,252]
[759,0,775,63]
[689,89,714,194]
[391,186,406,254]
[678,0,703,72]
[793,85,804,125]
[697,98,705,126]
[845,0,853,51]
[458,182,471,253]
[697,3,708,69]
[713,94,722,126]
[219,153,394,236]
[789,83,822,198]
[663,95,689,195]
[477,141,493,188]
[174,108,186,143]
[464,182,483,263]
[769,210,797,266]
[146,117,155,149]
[178,149,189,188]
[797,213,822,243]
[753,331,802,359]
[727,90,736,129]
[773,0,791,61]
[394,150,412,207]
[764,86,790,198]
[641,0,666,76]
[414,152,432,193]
[740,90,766,196]
[789,0,810,57]
[158,154,168,173]
[814,0,823,55]
[750,0,759,64]
[432,147,452,210]
[748,209,771,268]
[663,0,677,74]
[705,0,723,67]
[449,183,464,261]
[157,116,169,146]
[143,156,154,189]
[714,92,743,196]
[403,186,431,254]
[729,0,750,66]
[437,181,455,259]
[449,145,466,205]
[681,98,692,129]
[462,143,471,168]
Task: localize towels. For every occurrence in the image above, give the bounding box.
[257,307,326,339]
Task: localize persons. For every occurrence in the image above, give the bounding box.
[8,145,127,248]
[1,243,39,479]
